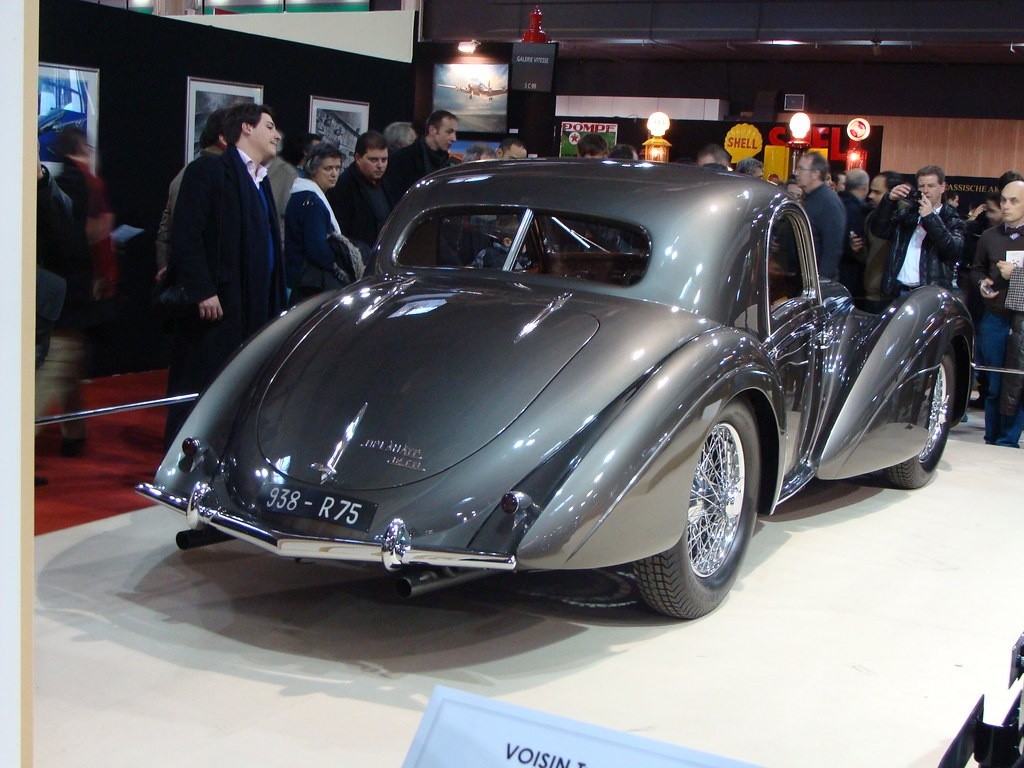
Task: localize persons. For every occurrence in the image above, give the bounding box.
[26,102,1024,488]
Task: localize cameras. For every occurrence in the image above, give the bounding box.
[905,190,922,202]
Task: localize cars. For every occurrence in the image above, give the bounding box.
[134,156,975,620]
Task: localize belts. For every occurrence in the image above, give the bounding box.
[902,284,920,292]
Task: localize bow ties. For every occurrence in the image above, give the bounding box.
[1007,226,1024,236]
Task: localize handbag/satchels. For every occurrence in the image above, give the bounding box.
[143,269,221,359]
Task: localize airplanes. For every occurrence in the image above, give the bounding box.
[438,79,508,100]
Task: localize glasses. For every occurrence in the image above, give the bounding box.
[84,143,95,153]
[794,166,821,174]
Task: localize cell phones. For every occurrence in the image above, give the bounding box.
[982,285,994,294]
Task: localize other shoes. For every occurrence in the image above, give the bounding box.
[35,475,48,484]
[970,399,985,407]
[62,438,85,457]
[961,414,968,422]
